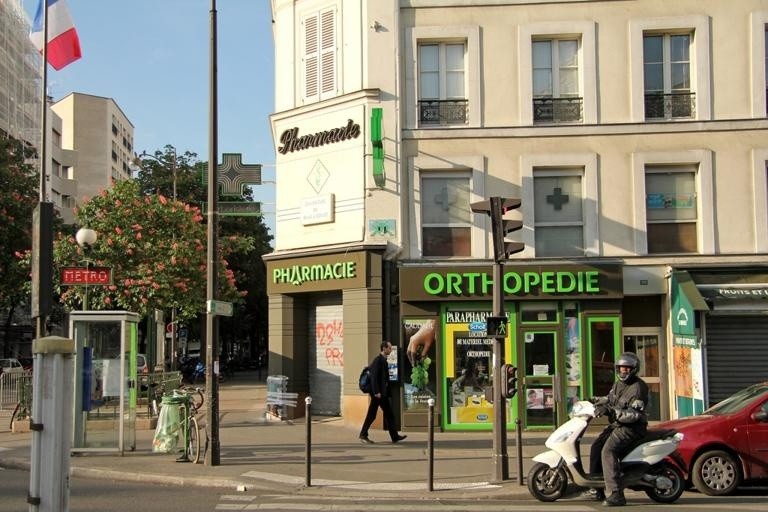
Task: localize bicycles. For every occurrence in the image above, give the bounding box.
[147,374,206,465]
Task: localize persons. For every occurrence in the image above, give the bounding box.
[579,351,650,506]
[453,368,470,393]
[404,317,436,369]
[357,340,408,445]
[526,390,543,408]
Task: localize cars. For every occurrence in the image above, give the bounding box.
[0,357,26,387]
[645,378,768,498]
[112,352,151,393]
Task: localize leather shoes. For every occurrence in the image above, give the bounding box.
[391,435,407,443]
[584,488,606,499]
[359,437,374,444]
[601,490,626,506]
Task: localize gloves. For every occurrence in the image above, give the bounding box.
[594,405,609,418]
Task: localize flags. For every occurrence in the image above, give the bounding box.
[28,1,81,74]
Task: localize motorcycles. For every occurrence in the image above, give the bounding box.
[525,394,689,506]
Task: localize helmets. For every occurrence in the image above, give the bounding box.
[615,353,640,383]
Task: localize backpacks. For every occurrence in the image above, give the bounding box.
[359,366,371,393]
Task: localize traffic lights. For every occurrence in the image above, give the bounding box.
[468,192,524,486]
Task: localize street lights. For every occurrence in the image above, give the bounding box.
[73,219,105,456]
